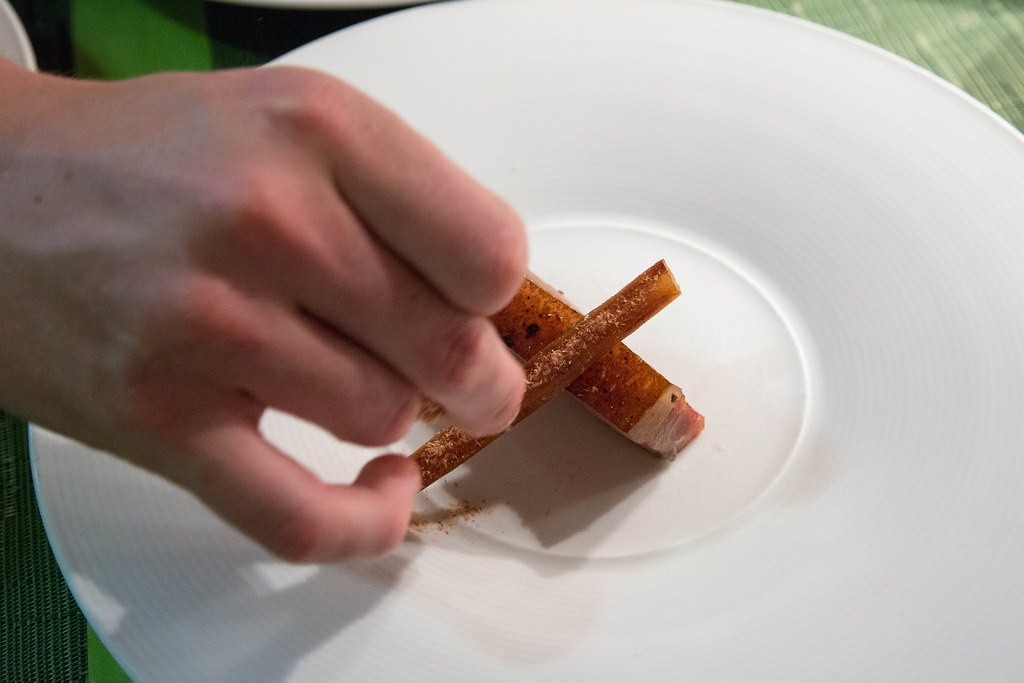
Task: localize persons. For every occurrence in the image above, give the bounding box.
[2,2,528,563]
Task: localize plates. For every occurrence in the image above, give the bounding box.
[29,0,1024,683]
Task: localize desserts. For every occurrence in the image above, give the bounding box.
[403,258,706,493]
